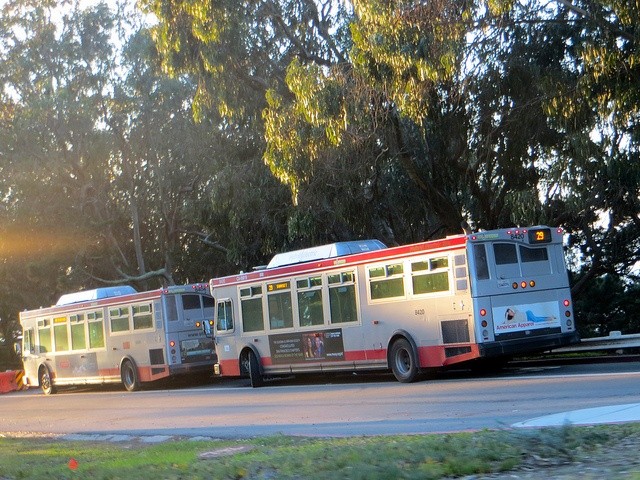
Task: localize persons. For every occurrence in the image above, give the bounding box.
[303,292,320,326]
[314,335,324,357]
[306,336,315,358]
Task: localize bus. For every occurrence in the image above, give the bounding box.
[202,225,578,387]
[14,283,217,394]
